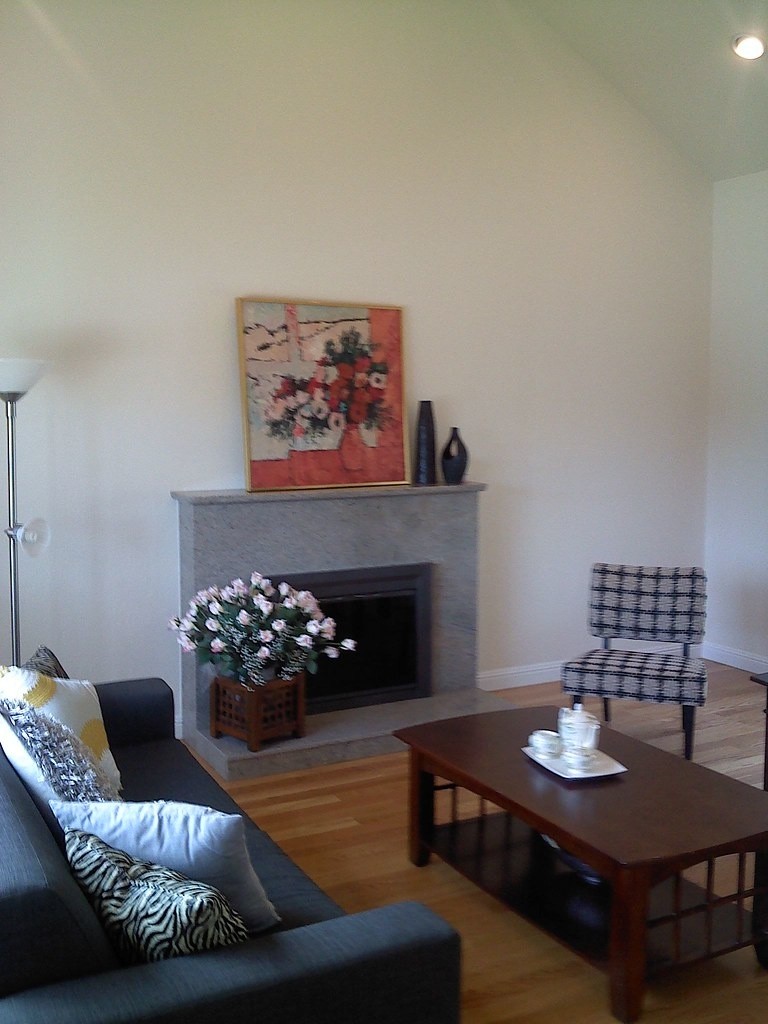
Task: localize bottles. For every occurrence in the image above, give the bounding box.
[441,427,467,485]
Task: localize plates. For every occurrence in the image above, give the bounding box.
[521,746,629,779]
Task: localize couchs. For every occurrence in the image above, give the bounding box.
[1,678,462,1024]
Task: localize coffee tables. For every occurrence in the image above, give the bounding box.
[391,706,768,1024]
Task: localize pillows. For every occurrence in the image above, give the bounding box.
[64,827,249,967]
[1,666,122,792]
[0,698,122,847]
[49,800,282,934]
[21,645,70,680]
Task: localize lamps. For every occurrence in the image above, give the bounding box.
[1,359,50,667]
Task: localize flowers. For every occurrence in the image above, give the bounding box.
[166,571,357,693]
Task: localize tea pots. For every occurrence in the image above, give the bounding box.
[558,704,601,747]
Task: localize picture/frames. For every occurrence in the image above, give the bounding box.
[236,296,413,493]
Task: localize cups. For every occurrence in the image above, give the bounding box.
[560,746,598,772]
[527,730,560,759]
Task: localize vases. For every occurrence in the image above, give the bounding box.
[441,427,469,485]
[224,663,278,685]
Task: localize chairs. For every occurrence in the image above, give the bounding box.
[561,563,708,761]
